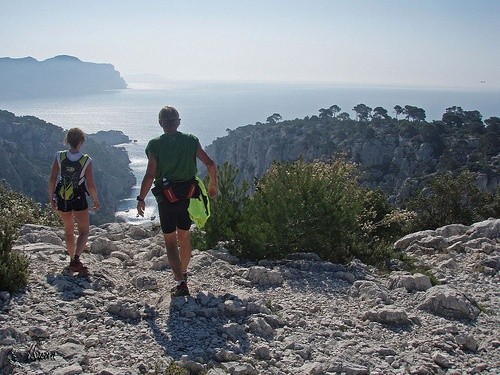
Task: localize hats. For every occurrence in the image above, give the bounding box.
[158,106,179,121]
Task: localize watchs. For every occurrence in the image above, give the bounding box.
[137,196,145,202]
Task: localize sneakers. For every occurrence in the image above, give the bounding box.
[174,273,188,282]
[171,281,191,296]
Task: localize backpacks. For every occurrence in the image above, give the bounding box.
[54,150,90,201]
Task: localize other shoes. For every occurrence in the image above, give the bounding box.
[66,259,88,273]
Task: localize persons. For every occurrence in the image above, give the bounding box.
[137,106,217,298]
[49,127,100,271]
